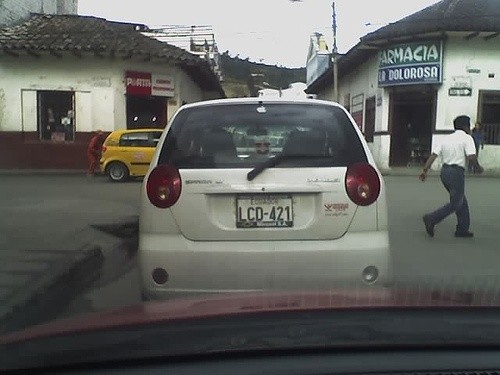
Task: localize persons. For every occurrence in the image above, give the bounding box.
[467,122,484,173]
[419,115,483,237]
[44,106,62,139]
[86,130,103,177]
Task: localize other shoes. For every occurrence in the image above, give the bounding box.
[455,232,473,237]
[423,214,434,236]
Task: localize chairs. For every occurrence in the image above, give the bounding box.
[200,128,245,166]
[279,129,325,166]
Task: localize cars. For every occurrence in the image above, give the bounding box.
[98,128,164,183]
[232,131,285,155]
[138,98,391,302]
